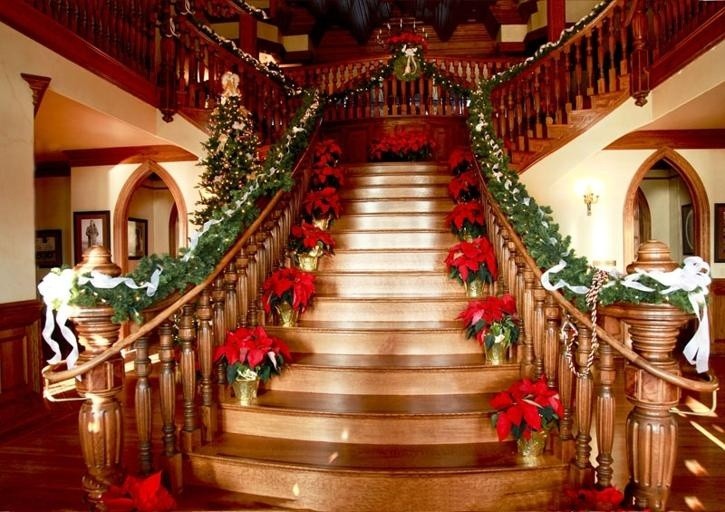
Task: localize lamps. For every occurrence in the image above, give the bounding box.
[572,179,602,217]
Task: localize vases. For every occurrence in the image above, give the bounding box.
[275,300,299,328]
[229,366,261,406]
[517,437,549,469]
[482,335,508,365]
[464,279,487,300]
[292,246,317,270]
[459,227,476,245]
[311,214,332,249]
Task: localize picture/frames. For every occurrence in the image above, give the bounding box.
[72,209,111,268]
[681,202,694,256]
[127,217,148,260]
[713,203,725,263]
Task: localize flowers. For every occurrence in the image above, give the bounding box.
[444,237,496,279]
[384,33,430,58]
[308,164,344,188]
[300,187,340,219]
[489,377,567,442]
[284,220,333,254]
[443,198,484,227]
[450,170,476,196]
[259,266,319,312]
[214,326,292,383]
[448,143,473,167]
[313,139,342,167]
[456,293,518,347]
[364,126,433,157]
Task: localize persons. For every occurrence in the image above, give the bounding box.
[85,220,99,248]
